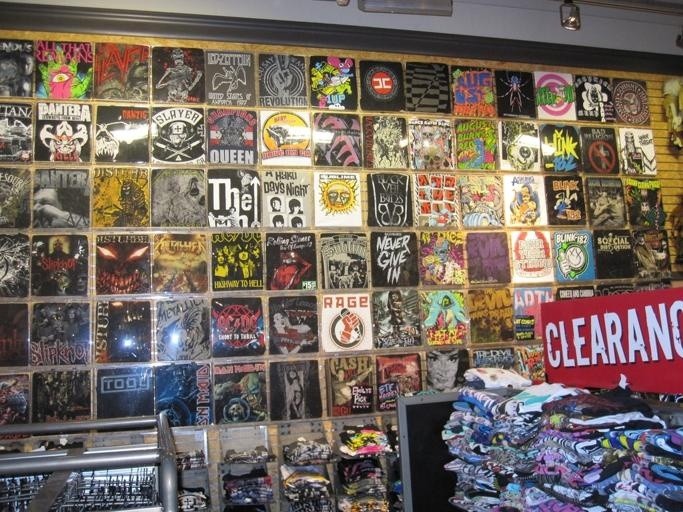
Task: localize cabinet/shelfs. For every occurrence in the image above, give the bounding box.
[0,412,404,512]
